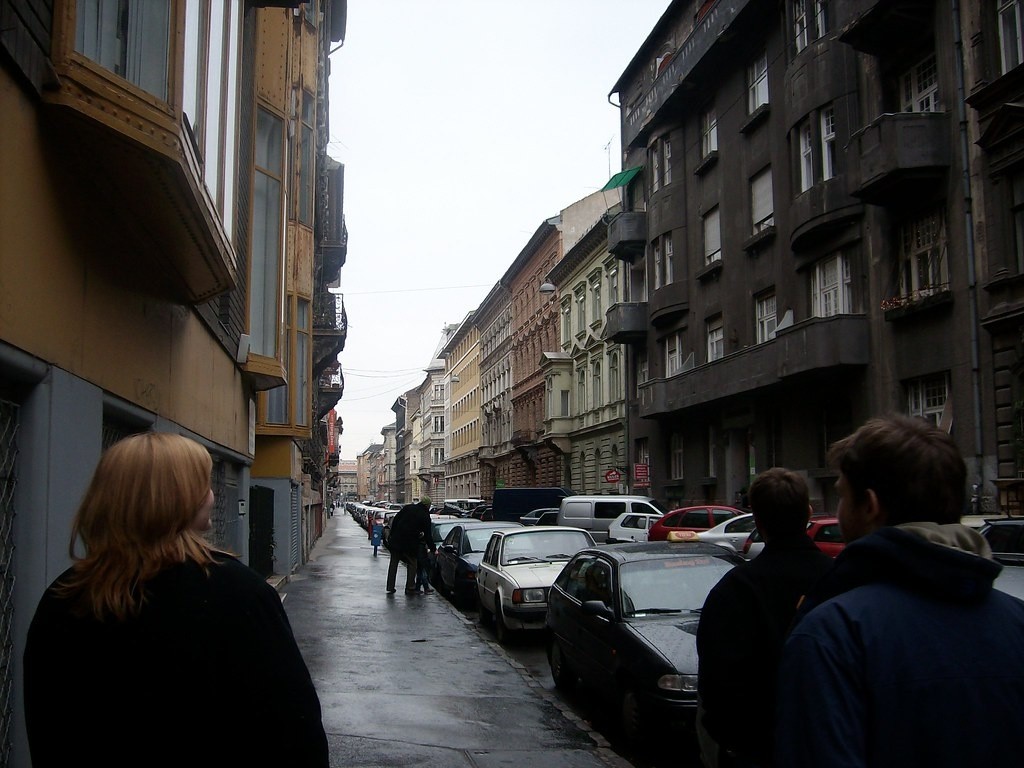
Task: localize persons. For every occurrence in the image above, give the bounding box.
[386,496,439,594]
[778,414,1024,768]
[23,433,330,768]
[696,466,844,768]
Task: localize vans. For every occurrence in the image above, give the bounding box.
[558,496,662,546]
[492,486,577,522]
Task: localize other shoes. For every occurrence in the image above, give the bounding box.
[417,590,423,594]
[424,588,434,594]
[387,587,396,592]
[405,589,420,595]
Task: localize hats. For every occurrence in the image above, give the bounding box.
[421,496,431,509]
[417,531,424,540]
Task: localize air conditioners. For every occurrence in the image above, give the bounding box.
[493,402,499,411]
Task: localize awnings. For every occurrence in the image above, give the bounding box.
[600,165,644,218]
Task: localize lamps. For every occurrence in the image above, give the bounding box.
[451,374,460,383]
[538,278,556,296]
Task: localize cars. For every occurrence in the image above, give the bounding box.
[805,517,846,560]
[437,522,525,609]
[960,514,1024,604]
[544,542,746,768]
[647,504,747,542]
[605,513,666,544]
[696,514,766,563]
[477,527,598,646]
[520,508,559,527]
[345,499,492,589]
